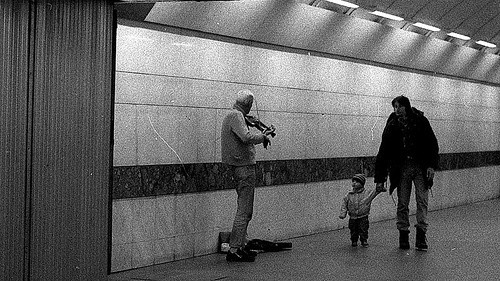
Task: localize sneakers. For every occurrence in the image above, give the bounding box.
[240,247,257,256]
[226,248,255,262]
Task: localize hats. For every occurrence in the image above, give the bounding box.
[352,174,365,185]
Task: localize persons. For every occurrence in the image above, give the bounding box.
[220,89,276,263]
[373,95,439,252]
[338,173,388,249]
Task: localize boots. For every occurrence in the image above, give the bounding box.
[399,230,410,249]
[415,228,428,249]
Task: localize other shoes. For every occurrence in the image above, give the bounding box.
[352,242,357,246]
[362,240,369,248]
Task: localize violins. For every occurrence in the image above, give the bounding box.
[245,115,276,138]
